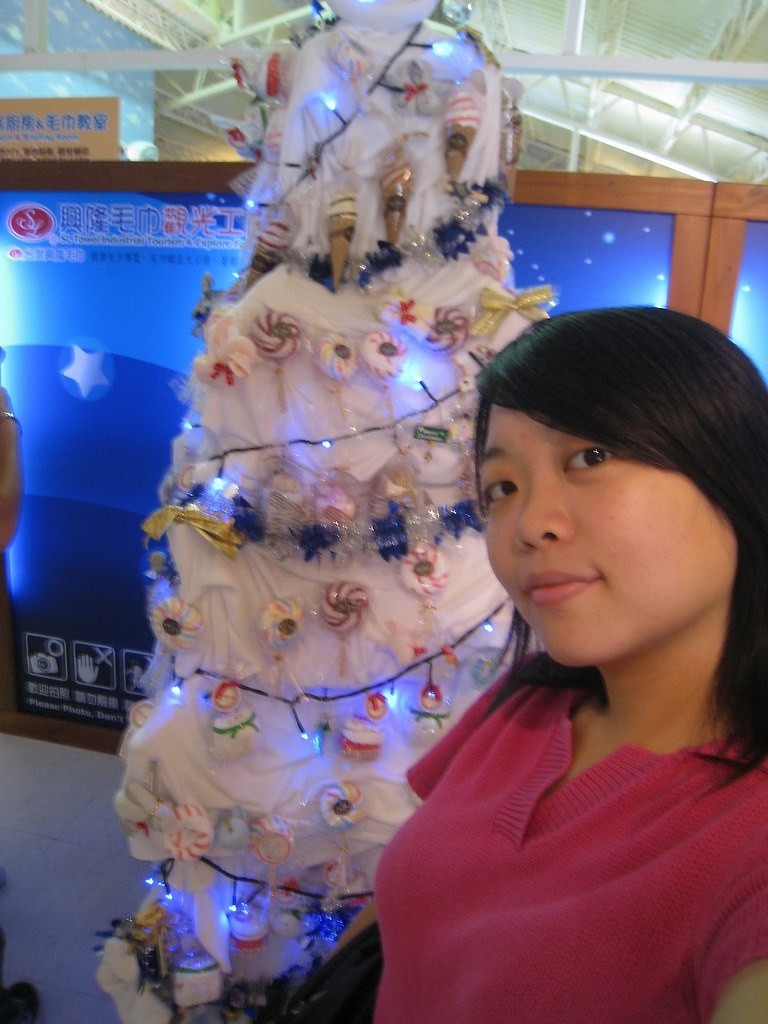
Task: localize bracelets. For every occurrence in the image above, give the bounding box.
[0,411,20,425]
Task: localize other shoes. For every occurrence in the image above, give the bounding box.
[0,981,39,1024]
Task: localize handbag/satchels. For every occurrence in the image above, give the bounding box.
[282,922,383,1024]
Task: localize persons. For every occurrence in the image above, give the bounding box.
[0,386,41,1024]
[323,305,768,1024]
